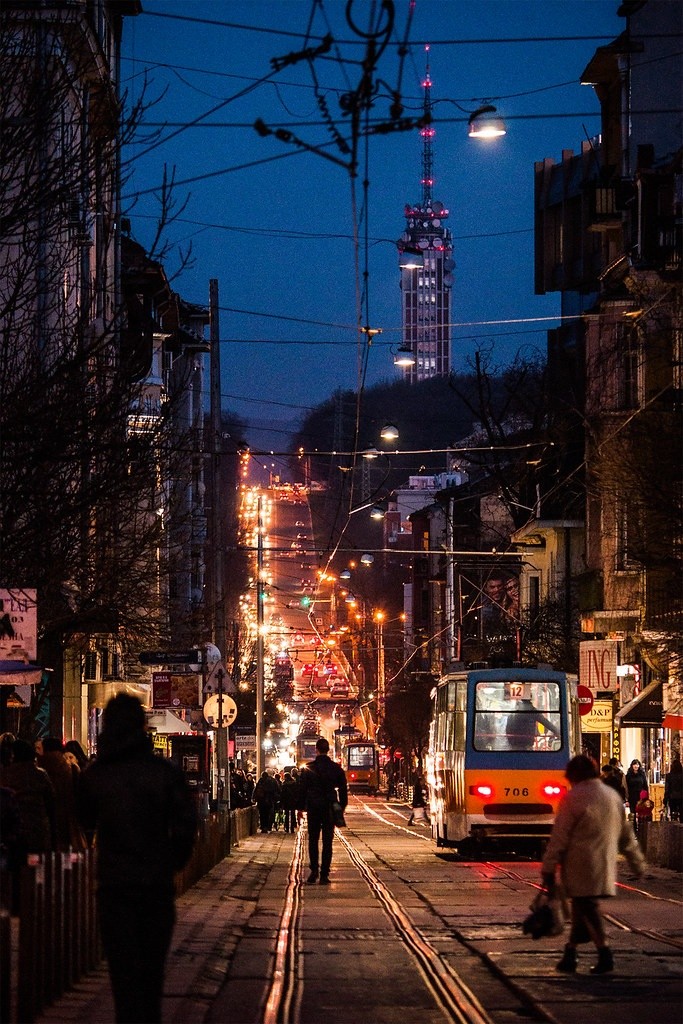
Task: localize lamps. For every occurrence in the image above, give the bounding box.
[361,553,374,563]
[380,425,399,439]
[371,504,384,518]
[398,242,425,269]
[468,99,506,137]
[362,448,379,458]
[345,593,355,603]
[339,570,351,578]
[393,344,416,365]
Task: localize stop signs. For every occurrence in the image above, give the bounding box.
[577,685,594,716]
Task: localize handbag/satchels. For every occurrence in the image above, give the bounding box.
[329,800,347,828]
[521,879,565,940]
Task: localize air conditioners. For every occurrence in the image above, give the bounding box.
[81,650,119,683]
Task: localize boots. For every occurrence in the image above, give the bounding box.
[407,820,415,826]
[591,944,613,975]
[557,943,576,973]
[428,819,431,826]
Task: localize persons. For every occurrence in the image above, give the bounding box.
[76,695,200,1023]
[477,698,561,751]
[229,763,256,810]
[368,768,378,796]
[601,757,626,801]
[540,754,649,976]
[408,777,432,828]
[625,758,648,812]
[386,772,398,801]
[253,765,307,834]
[0,731,96,902]
[635,789,654,824]
[662,760,683,824]
[298,740,349,885]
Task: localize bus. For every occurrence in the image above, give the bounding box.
[340,743,380,795]
[424,667,573,852]
[288,735,322,771]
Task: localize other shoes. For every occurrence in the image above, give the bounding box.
[306,869,318,884]
[320,876,332,886]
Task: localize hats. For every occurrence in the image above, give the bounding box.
[640,790,649,801]
